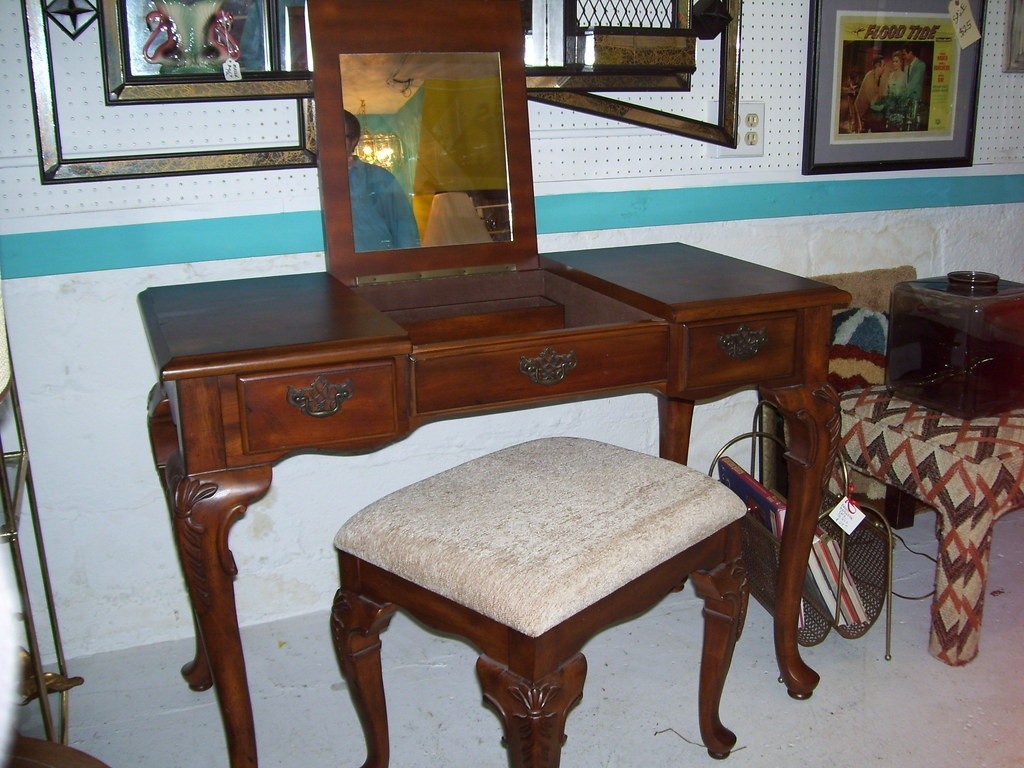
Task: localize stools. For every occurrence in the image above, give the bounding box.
[329,438,752,768]
[784,381,1024,666]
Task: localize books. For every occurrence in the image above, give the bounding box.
[719,455,805,629]
[775,490,869,627]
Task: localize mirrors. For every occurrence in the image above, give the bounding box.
[336,52,515,254]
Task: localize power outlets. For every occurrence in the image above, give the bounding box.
[704,101,764,157]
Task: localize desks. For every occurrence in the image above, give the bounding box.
[135,241,852,768]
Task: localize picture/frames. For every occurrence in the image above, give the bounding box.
[802,0,988,174]
[97,0,695,106]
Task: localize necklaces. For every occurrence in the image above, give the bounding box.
[873,71,882,88]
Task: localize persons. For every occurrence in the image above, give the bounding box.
[903,44,926,100]
[853,54,888,133]
[345,110,421,252]
[887,52,908,96]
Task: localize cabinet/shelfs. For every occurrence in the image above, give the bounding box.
[0,293,83,748]
